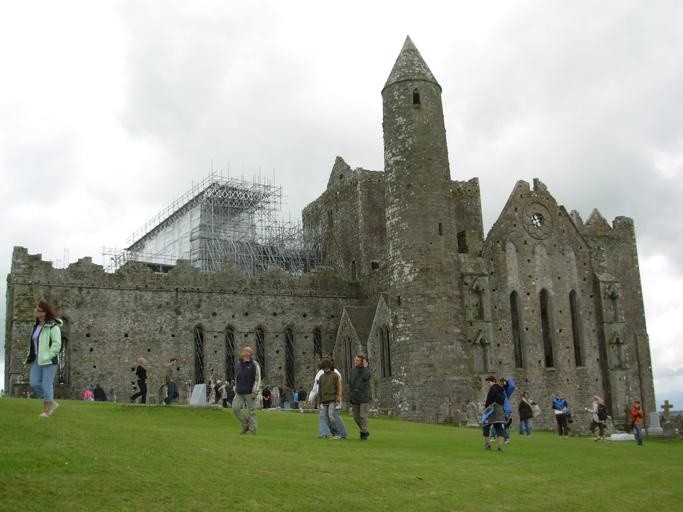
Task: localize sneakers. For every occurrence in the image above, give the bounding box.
[48,403,58,416]
[240,427,248,434]
[245,431,256,434]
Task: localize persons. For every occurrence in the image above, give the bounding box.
[84,383,107,402]
[518,391,532,438]
[260,385,307,413]
[552,391,568,438]
[308,350,373,440]
[130,356,177,405]
[584,396,606,442]
[24,299,63,415]
[207,379,236,409]
[231,347,260,436]
[480,376,516,453]
[629,401,644,446]
